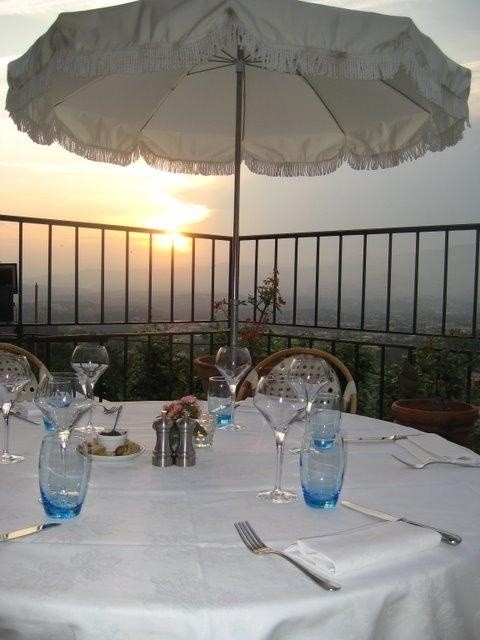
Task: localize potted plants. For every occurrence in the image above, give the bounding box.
[391,325,478,450]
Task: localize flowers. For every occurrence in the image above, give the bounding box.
[211,268,287,360]
[164,395,203,421]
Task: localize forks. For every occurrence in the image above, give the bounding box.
[394,456,477,474]
[93,404,117,415]
[232,520,341,592]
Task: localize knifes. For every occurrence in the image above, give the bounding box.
[340,501,461,546]
[1,523,60,543]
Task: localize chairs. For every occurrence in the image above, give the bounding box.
[2,342,59,403]
[235,347,358,413]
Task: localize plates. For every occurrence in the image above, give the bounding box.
[74,440,145,461]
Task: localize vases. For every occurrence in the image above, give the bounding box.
[192,350,268,397]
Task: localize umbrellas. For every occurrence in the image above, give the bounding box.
[4,0,472,369]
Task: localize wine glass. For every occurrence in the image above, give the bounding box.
[35,372,93,499]
[71,344,109,433]
[253,375,307,505]
[289,357,329,455]
[215,346,252,432]
[0,355,33,464]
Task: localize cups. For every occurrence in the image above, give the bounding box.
[309,392,341,450]
[208,376,234,428]
[38,434,92,518]
[189,412,217,448]
[300,430,347,510]
[96,428,127,451]
